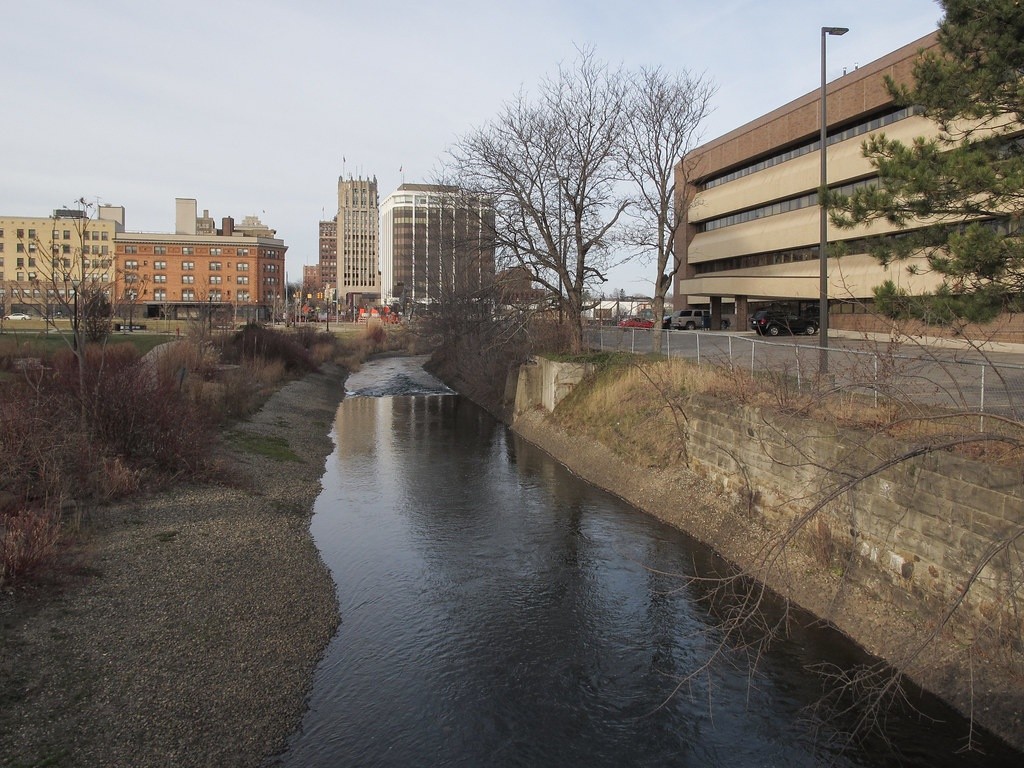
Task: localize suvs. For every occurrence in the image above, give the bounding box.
[670,309,731,331]
[750,307,819,337]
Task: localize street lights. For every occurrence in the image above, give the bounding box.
[72,272,79,358]
[812,26,850,383]
[559,177,572,326]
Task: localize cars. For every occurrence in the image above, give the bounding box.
[3,313,32,321]
[618,316,655,328]
[663,315,671,330]
